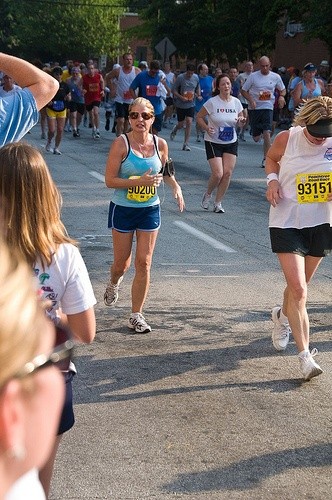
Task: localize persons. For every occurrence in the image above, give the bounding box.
[0,240,75,500]
[40,53,254,154]
[0,137,97,496]
[0,52,60,148]
[241,56,286,168]
[264,96,332,381]
[270,60,332,138]
[196,74,247,213]
[103,97,185,334]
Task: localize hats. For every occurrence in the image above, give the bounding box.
[305,118,331,138]
[303,64,315,71]
[320,61,329,67]
[71,68,81,74]
[113,64,120,70]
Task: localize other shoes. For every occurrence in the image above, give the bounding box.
[96,131,101,138]
[262,159,265,166]
[112,128,116,133]
[196,137,201,142]
[239,134,246,141]
[170,125,178,141]
[54,148,62,155]
[63,120,93,137]
[183,144,190,151]
[46,141,51,152]
[105,120,110,131]
[41,132,46,139]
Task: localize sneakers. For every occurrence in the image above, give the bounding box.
[128,312,151,332]
[299,347,321,379]
[104,275,124,305]
[271,307,291,350]
[213,201,225,213]
[201,192,212,209]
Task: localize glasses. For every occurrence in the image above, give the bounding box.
[0,323,73,398]
[129,111,154,120]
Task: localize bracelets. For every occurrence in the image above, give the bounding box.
[267,173,279,185]
